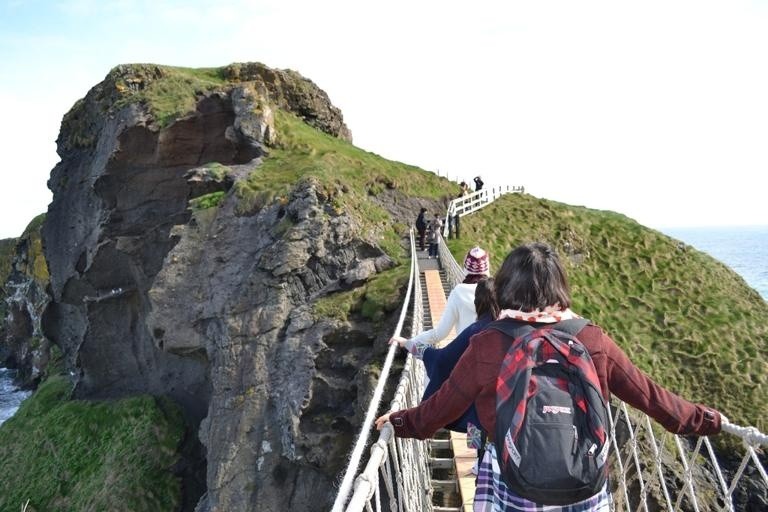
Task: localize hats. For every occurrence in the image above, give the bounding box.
[420,207,427,213]
[464,246,490,277]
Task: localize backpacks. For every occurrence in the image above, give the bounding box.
[476,316,612,505]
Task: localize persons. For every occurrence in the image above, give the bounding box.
[374,243,730,512]
[399,277,501,485]
[388,246,490,353]
[416,208,428,251]
[459,182,470,210]
[473,176,484,206]
[428,213,442,259]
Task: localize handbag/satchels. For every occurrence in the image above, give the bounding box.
[458,193,464,198]
[426,232,436,242]
